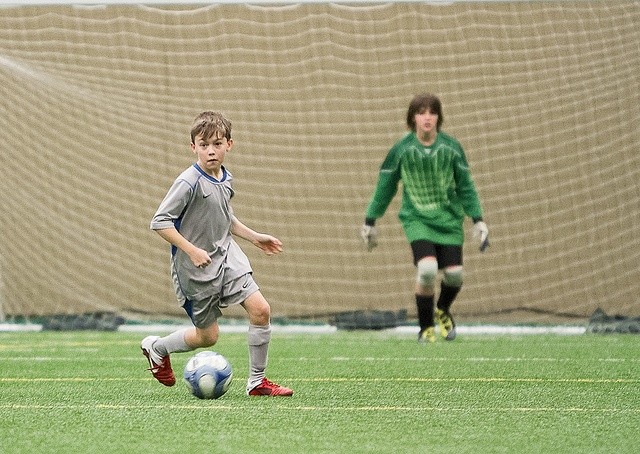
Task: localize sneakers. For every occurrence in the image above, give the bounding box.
[140,335,175,386]
[246,376,294,396]
[420,327,436,342]
[434,307,456,340]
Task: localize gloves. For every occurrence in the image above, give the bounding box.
[473,221,492,253]
[362,224,377,253]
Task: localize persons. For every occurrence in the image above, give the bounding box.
[139,112,294,395]
[361,92,491,342]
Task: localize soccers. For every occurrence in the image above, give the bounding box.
[184,351,233,399]
